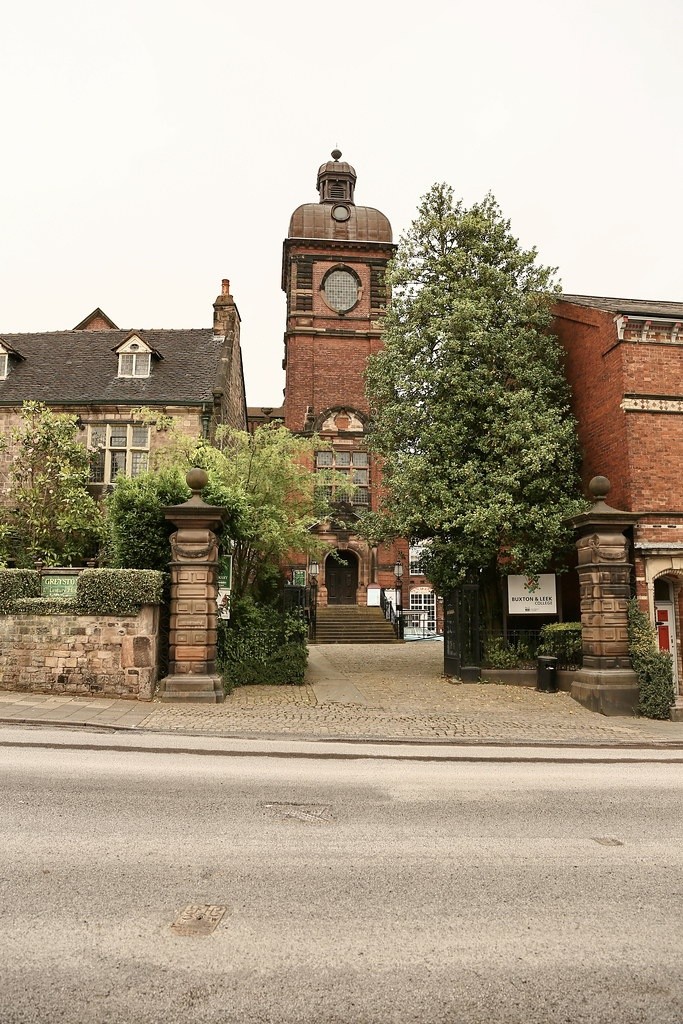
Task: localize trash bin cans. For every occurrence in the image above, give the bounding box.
[536,656,559,693]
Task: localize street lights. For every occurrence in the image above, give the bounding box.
[394,557,403,638]
[309,559,320,640]
